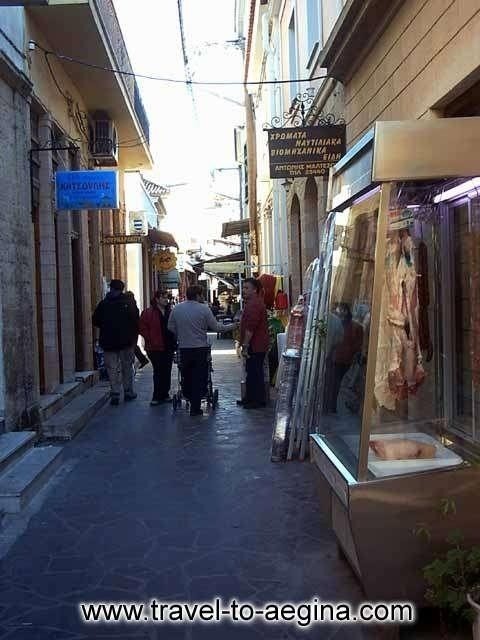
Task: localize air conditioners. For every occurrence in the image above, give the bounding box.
[91,119,119,167]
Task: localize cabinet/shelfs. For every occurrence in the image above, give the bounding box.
[307,114,479,605]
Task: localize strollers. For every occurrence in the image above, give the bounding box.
[171,339,218,410]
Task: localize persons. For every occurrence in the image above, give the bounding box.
[91,277,269,415]
[325,301,361,411]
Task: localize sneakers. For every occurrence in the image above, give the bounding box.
[190,409,203,417]
[109,398,119,405]
[150,395,173,406]
[236,398,265,409]
[124,392,137,401]
